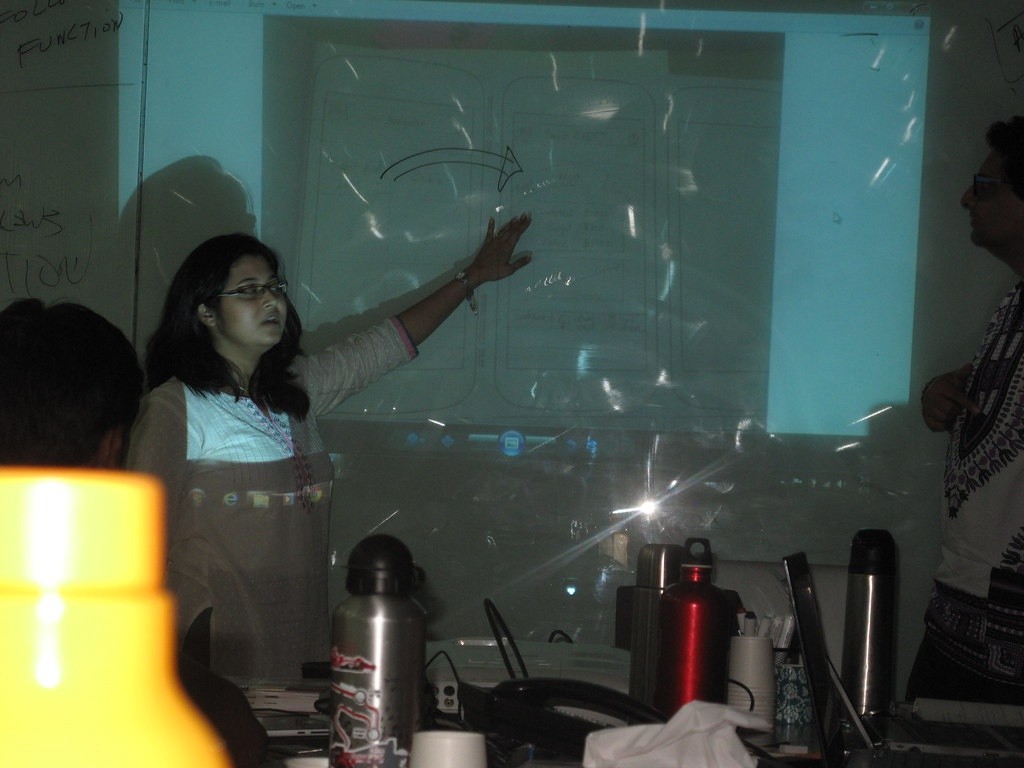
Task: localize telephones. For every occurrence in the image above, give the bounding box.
[461,676,667,768]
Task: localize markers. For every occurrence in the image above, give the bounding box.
[733,605,794,662]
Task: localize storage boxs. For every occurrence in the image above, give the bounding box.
[422,634,632,720]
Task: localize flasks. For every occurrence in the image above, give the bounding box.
[629,544,682,710]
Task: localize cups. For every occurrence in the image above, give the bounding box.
[728,635,775,733]
[412,732,485,768]
[776,663,815,727]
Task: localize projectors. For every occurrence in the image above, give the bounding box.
[423,636,632,715]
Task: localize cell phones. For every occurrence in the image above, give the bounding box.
[258,716,330,738]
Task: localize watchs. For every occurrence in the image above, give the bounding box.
[455,272,478,313]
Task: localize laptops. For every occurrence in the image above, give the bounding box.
[783,552,1024,768]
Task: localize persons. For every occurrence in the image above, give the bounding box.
[124,213,531,680]
[905,116,1024,705]
[0,297,270,768]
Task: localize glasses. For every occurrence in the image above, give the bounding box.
[219,278,289,297]
[972,173,1015,200]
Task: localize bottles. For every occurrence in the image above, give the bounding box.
[328,536,421,768]
[840,529,896,716]
[659,538,731,720]
